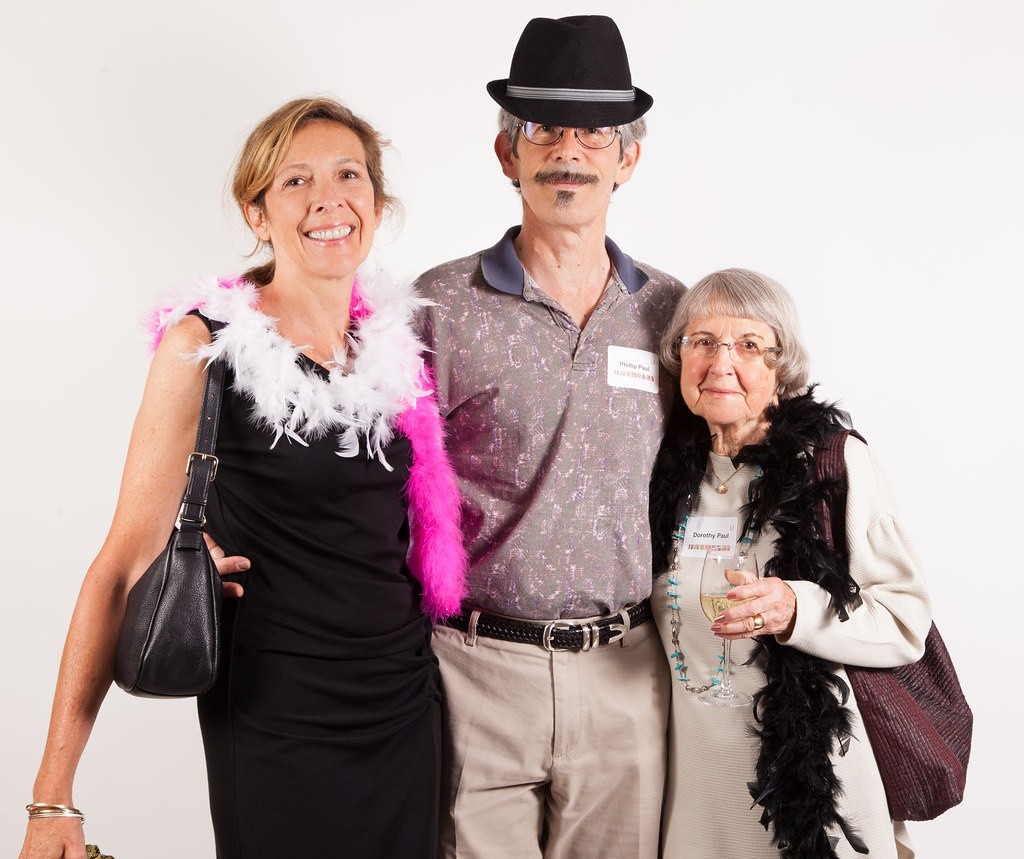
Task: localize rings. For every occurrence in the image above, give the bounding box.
[751,614,764,630]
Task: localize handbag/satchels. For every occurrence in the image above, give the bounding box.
[111,310,223,700]
[812,425,976,824]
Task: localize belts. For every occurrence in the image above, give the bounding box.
[436,597,650,655]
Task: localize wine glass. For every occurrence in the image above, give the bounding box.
[697,549,759,707]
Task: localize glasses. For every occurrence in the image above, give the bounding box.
[677,335,783,364]
[505,121,622,150]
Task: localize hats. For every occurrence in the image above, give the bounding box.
[484,14,655,130]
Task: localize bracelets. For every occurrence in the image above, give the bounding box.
[26,802,85,825]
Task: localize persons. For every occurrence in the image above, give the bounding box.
[18,98,460,859]
[645,267,934,859]
[201,14,691,859]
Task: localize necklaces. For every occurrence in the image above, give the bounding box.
[665,470,765,694]
[708,456,745,494]
[142,267,433,451]
